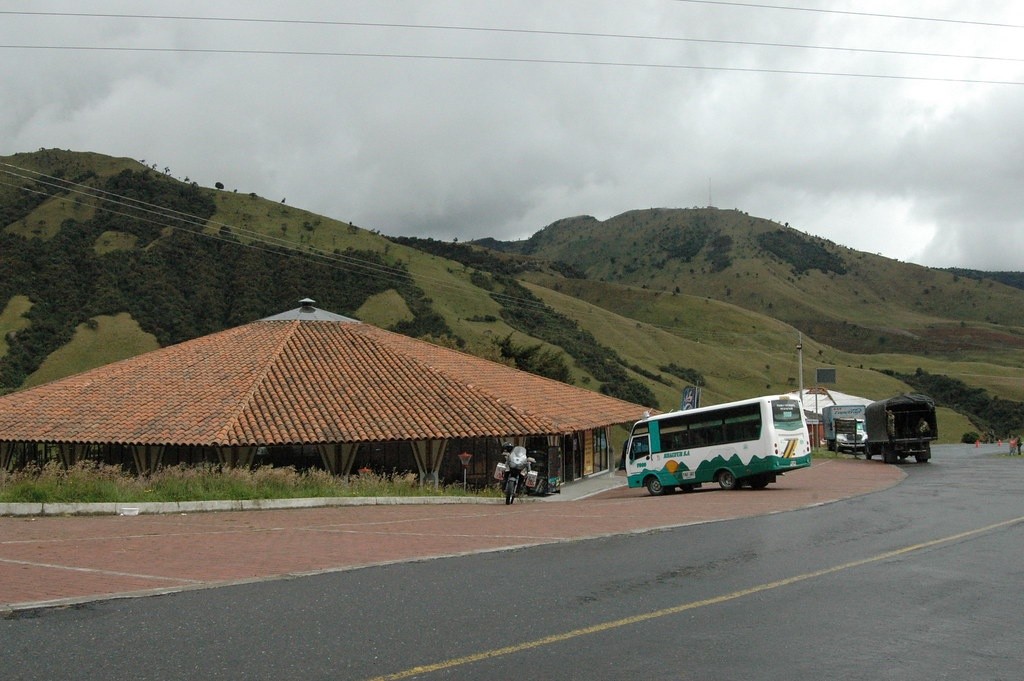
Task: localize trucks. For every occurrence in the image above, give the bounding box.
[864,395,938,464]
[822,405,867,452]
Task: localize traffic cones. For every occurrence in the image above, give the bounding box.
[998,439,1003,446]
[975,439,980,448]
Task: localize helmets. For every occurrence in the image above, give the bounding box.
[502,441,514,453]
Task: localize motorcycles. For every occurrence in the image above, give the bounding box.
[496,440,537,506]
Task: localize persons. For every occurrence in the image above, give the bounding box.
[1009,436,1022,455]
[885,410,896,443]
[916,418,930,438]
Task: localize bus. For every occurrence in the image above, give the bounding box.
[622,390,813,493]
